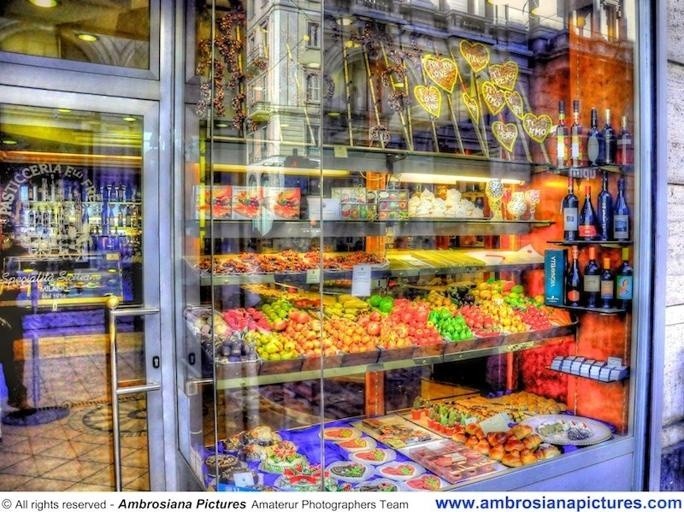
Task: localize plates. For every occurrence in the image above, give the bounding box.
[523,414,612,450]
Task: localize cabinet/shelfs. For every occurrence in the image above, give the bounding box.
[170,1,653,492]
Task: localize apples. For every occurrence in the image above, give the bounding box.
[196,279,552,362]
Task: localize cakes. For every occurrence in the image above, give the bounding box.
[500,391,567,414]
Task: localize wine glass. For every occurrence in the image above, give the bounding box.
[485,177,542,223]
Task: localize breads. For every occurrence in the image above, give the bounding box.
[452,423,561,468]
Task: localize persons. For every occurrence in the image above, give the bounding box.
[0,212,36,414]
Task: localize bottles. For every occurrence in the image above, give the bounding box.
[23,175,140,252]
[553,100,634,312]
